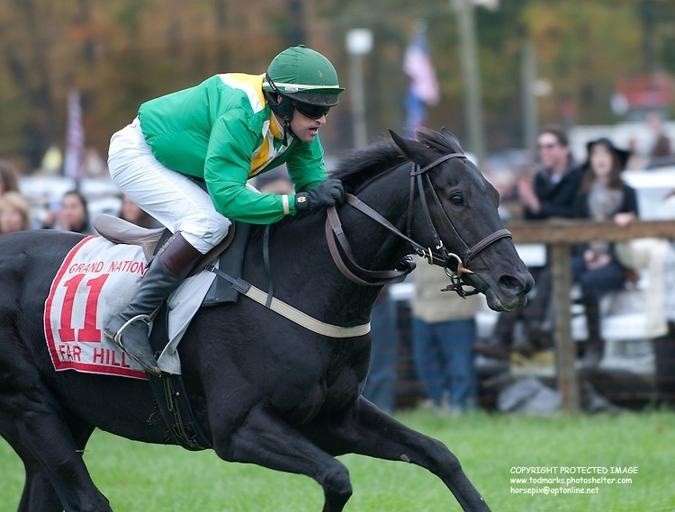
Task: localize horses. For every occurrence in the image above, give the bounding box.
[0,124,536,512]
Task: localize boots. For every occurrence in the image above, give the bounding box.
[104,233,204,377]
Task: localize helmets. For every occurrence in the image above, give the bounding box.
[263,44,344,106]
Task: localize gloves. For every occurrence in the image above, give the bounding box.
[296,179,345,210]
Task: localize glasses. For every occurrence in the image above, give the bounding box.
[295,100,329,119]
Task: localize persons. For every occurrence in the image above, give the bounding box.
[367,112,672,419]
[0,161,154,236]
[103,45,345,382]
